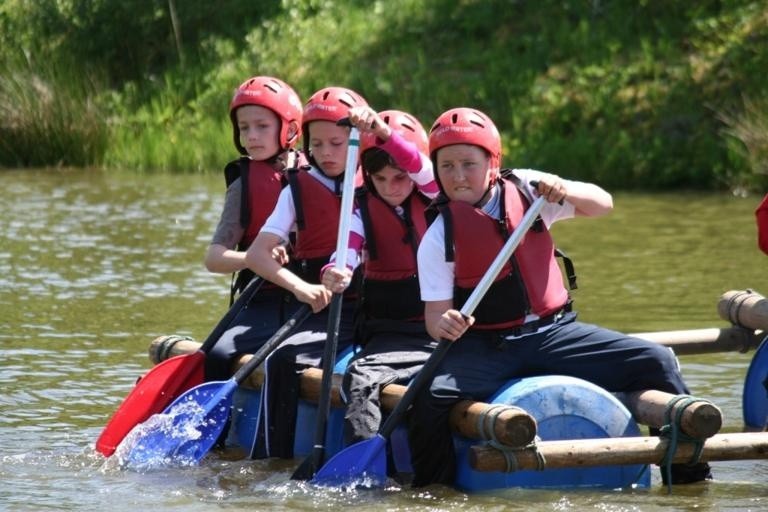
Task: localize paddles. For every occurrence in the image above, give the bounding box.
[288,118,375,483]
[129,301,309,465]
[95,275,266,456]
[315,180,563,486]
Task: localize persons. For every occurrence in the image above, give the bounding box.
[243,86,369,460]
[405,106,713,489]
[205,74,309,451]
[318,106,442,476]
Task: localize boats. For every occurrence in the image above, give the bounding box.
[149,289,768,494]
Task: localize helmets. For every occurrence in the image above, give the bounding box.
[230,76,501,160]
